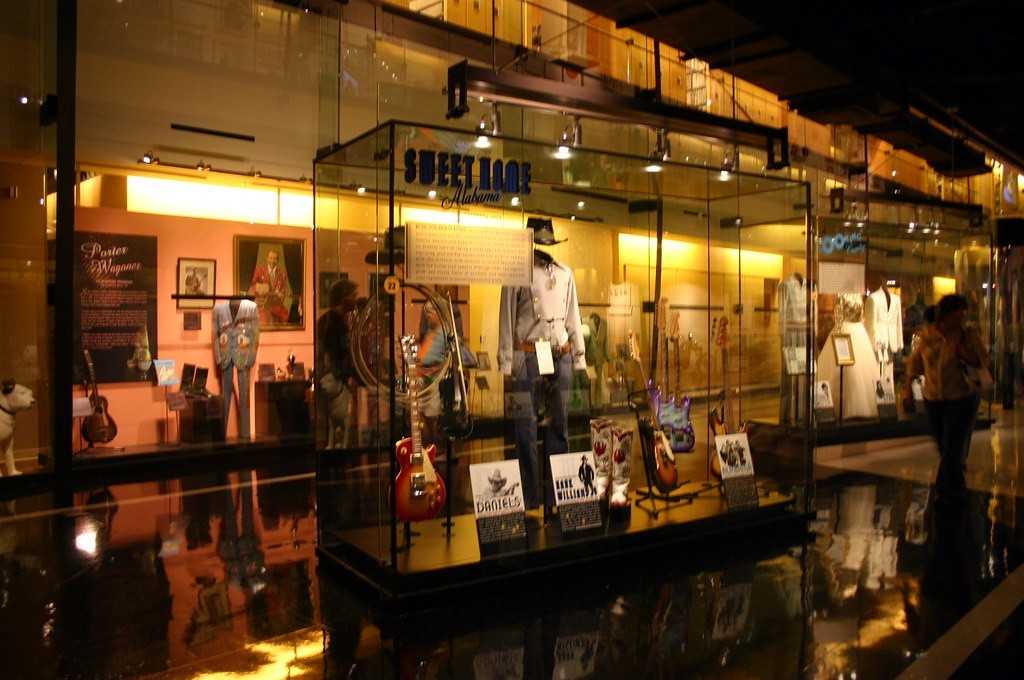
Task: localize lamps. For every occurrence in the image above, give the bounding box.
[641,128,672,173]
[551,114,582,160]
[718,144,738,182]
[841,199,946,234]
[476,100,502,148]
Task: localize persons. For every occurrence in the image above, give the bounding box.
[818,383,831,406]
[866,278,905,361]
[211,293,261,443]
[582,311,610,414]
[317,276,474,443]
[495,213,586,526]
[577,455,596,499]
[875,381,886,400]
[721,440,747,470]
[249,247,292,322]
[814,292,880,420]
[777,275,820,429]
[905,293,990,511]
[475,468,520,504]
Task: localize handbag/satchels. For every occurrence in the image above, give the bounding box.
[959,356,993,392]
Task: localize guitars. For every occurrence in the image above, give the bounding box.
[78,350,119,443]
[387,336,449,520]
[657,310,697,452]
[239,283,291,306]
[625,328,677,492]
[648,296,670,425]
[710,316,749,480]
[185,273,208,295]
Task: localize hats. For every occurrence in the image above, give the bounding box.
[527,218,568,245]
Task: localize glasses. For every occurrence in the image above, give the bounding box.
[545,266,552,289]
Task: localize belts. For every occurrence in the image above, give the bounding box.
[513,341,571,357]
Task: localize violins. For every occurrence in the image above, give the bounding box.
[438,291,476,440]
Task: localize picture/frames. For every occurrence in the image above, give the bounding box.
[233,234,307,332]
[176,257,217,309]
[831,333,855,367]
[369,272,390,313]
[476,351,492,371]
[319,271,349,309]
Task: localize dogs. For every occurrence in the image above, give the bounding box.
[0,378,38,475]
